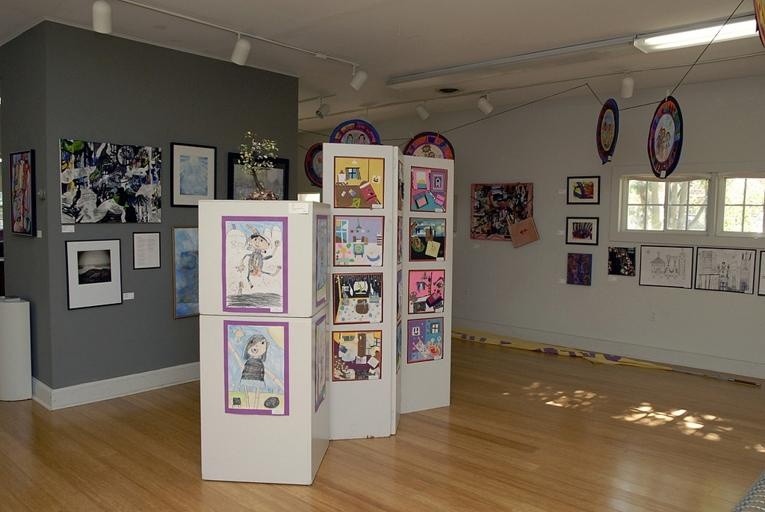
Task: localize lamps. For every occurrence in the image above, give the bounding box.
[91,0,367,91]
[316,95,329,119]
[415,76,635,122]
[631,13,760,56]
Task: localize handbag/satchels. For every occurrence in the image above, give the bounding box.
[508,217,538,248]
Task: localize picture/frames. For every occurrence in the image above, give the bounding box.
[170,226,198,320]
[133,230,161,270]
[169,141,217,208]
[638,244,765,297]
[228,153,289,201]
[9,149,36,238]
[567,175,602,205]
[566,216,600,245]
[65,238,123,313]
